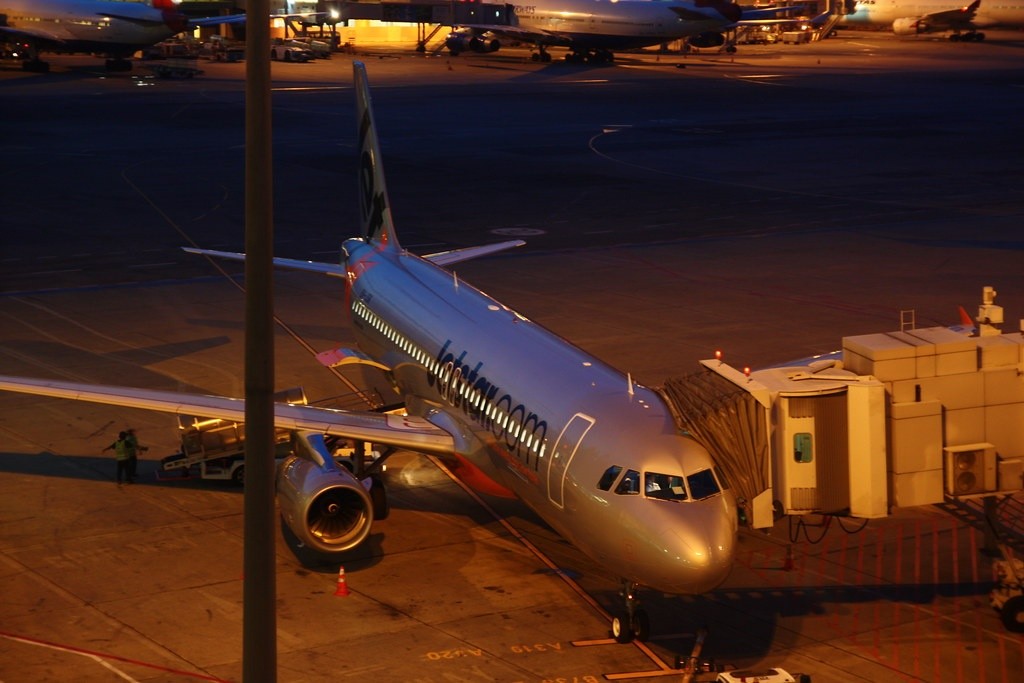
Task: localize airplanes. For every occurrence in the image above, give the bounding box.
[0,59,843,641]
[0,0,1024,72]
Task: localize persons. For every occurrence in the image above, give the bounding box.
[102,429,148,483]
[619,473,672,494]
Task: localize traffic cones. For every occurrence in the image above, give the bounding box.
[333,565,351,596]
